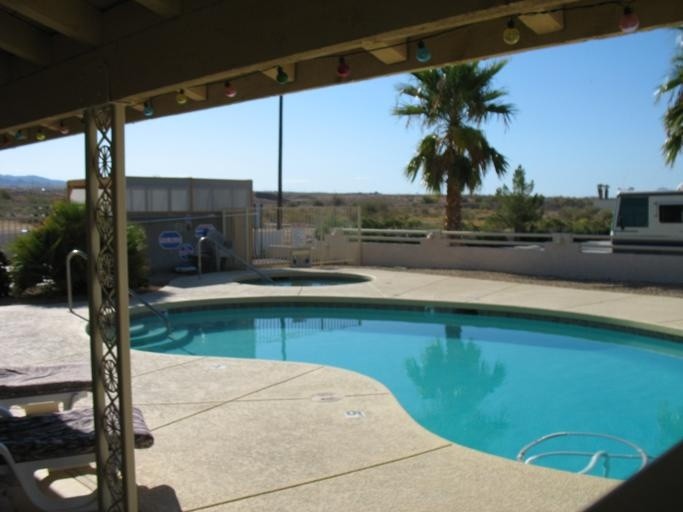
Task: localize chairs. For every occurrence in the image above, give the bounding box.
[1,363,154,512]
